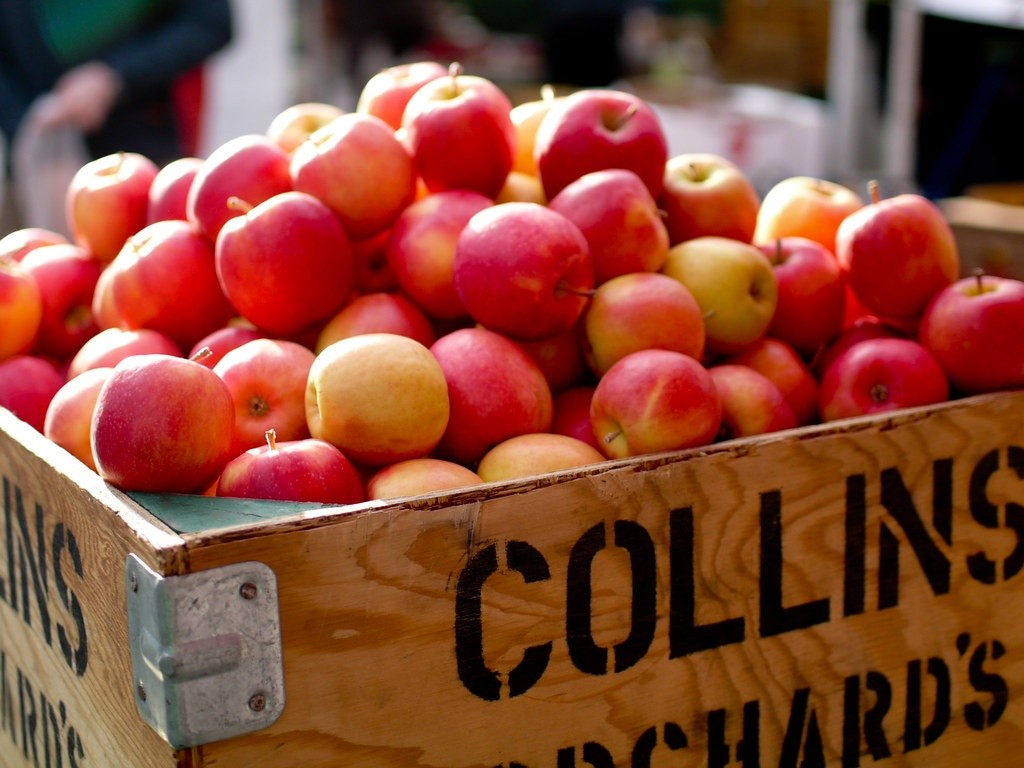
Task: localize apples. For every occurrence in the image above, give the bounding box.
[0,60,1024,504]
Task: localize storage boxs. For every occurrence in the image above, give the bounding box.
[0,204,1024,767]
